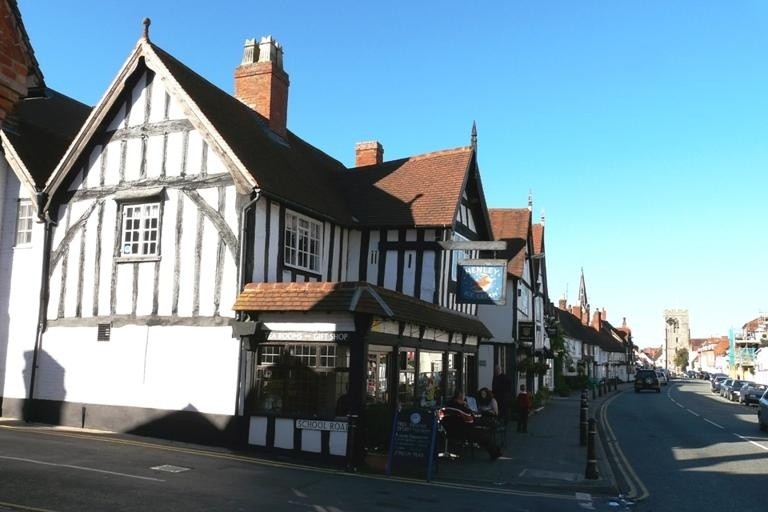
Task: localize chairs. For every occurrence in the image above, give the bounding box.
[488,400,506,444]
[441,416,476,462]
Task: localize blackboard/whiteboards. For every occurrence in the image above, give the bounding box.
[388,405,437,466]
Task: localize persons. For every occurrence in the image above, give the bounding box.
[444,388,512,462]
[516,384,532,433]
[492,365,510,425]
[479,388,498,422]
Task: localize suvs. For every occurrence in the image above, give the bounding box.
[634,370,661,392]
[657,371,668,384]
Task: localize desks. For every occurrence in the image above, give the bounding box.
[472,417,496,432]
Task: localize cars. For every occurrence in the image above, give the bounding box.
[668,369,767,436]
